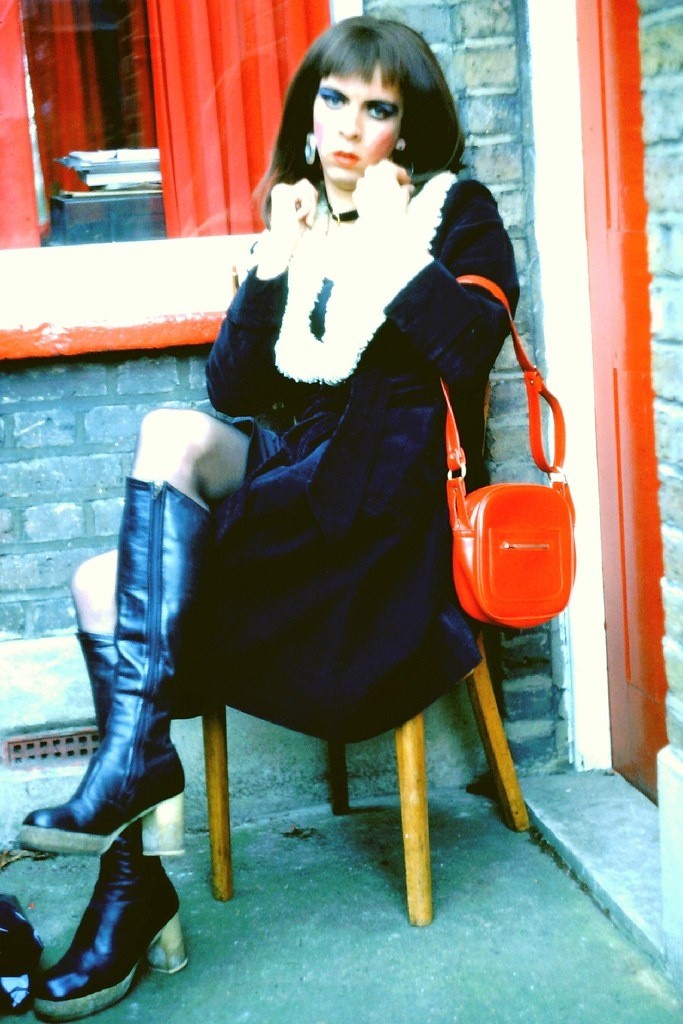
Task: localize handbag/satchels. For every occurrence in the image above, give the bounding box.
[439,271,576,626]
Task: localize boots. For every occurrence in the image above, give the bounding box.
[16,475,212,856]
[32,631,187,1022]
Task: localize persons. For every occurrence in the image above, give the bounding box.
[21,16,521,1024]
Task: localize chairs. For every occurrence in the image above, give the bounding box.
[203,379,531,927]
